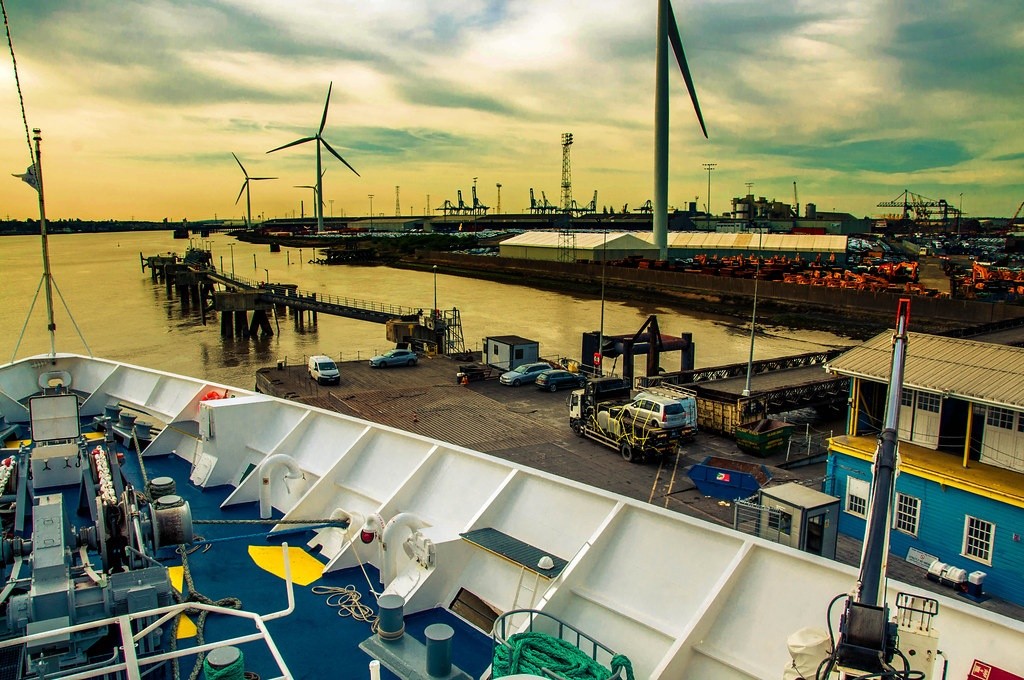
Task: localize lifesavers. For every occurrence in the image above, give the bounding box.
[198,391,220,413]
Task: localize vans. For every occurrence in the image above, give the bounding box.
[499,363,553,387]
[623,399,687,429]
[308,356,340,385]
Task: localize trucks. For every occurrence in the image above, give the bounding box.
[565,390,651,462]
[584,378,697,441]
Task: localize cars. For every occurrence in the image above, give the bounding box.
[848,238,911,263]
[918,238,1005,260]
[369,350,418,368]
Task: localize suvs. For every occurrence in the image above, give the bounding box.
[534,370,587,393]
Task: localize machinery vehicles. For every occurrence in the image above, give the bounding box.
[623,251,1024,300]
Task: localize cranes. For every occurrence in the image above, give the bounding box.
[877,189,955,207]
